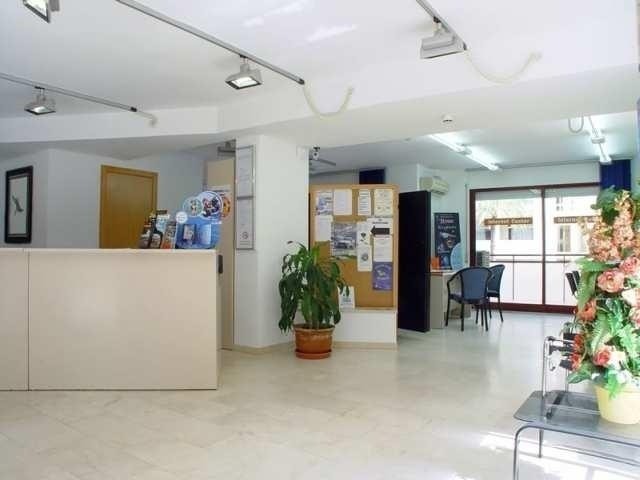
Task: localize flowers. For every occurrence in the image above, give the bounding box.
[562,184,640,391]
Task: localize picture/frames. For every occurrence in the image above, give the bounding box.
[5,164,33,243]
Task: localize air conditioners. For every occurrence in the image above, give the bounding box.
[420,176,450,196]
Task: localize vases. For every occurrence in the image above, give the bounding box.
[593,382,640,424]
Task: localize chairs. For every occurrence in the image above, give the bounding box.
[565,271,582,298]
[445,264,505,332]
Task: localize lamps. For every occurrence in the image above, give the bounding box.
[419,20,465,60]
[225,55,264,90]
[22,0,59,23]
[24,86,57,115]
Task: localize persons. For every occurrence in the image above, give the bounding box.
[437,228,459,267]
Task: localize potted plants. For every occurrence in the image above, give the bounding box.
[276,239,351,360]
